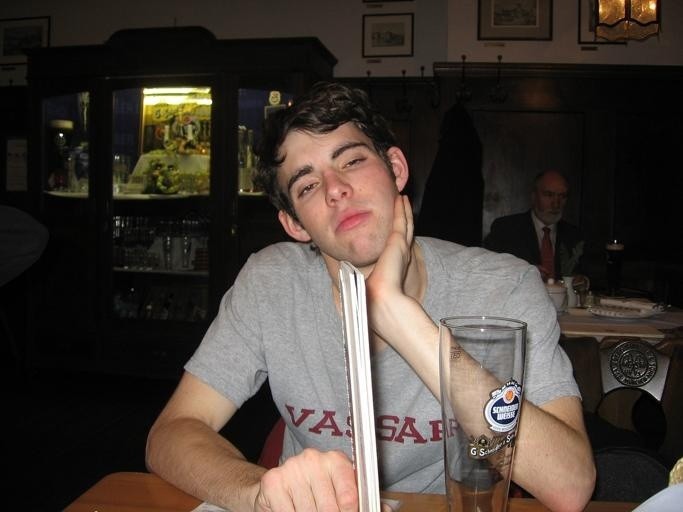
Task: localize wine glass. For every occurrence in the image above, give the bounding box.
[64,150,131,196]
[107,217,211,323]
[175,157,209,195]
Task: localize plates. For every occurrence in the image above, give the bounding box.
[588,304,668,320]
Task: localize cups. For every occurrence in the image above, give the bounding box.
[438,316,527,511]
[605,243,623,297]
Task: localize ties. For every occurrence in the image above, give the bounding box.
[537,226,558,280]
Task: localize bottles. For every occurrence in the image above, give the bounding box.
[238,124,263,193]
[47,118,75,191]
[545,274,596,311]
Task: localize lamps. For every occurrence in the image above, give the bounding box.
[590,0,662,45]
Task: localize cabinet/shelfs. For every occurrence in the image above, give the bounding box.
[24,28,339,382]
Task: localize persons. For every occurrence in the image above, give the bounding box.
[478,165,603,300]
[142,79,599,512]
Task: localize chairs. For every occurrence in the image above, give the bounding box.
[581,446,671,503]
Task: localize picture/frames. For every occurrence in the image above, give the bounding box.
[577,0,628,46]
[361,13,416,59]
[475,0,554,42]
